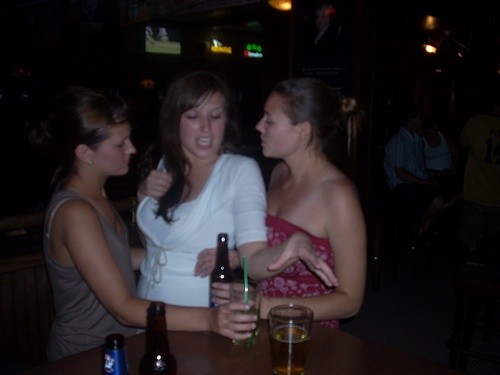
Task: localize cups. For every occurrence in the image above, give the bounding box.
[267,303,313,375]
[231,282,261,346]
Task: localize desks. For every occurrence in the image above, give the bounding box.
[35,321,443,375]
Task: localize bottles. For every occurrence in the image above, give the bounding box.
[103,334,126,375]
[130,199,138,223]
[209,233,233,307]
[138,302,177,375]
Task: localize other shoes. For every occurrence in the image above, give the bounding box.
[420,228,440,247]
[409,224,417,251]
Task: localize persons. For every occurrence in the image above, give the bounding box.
[43,86,258,362]
[314,5,333,44]
[137,78,367,328]
[0,96,500,242]
[135,70,339,308]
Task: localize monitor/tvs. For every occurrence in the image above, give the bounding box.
[145,26,182,54]
